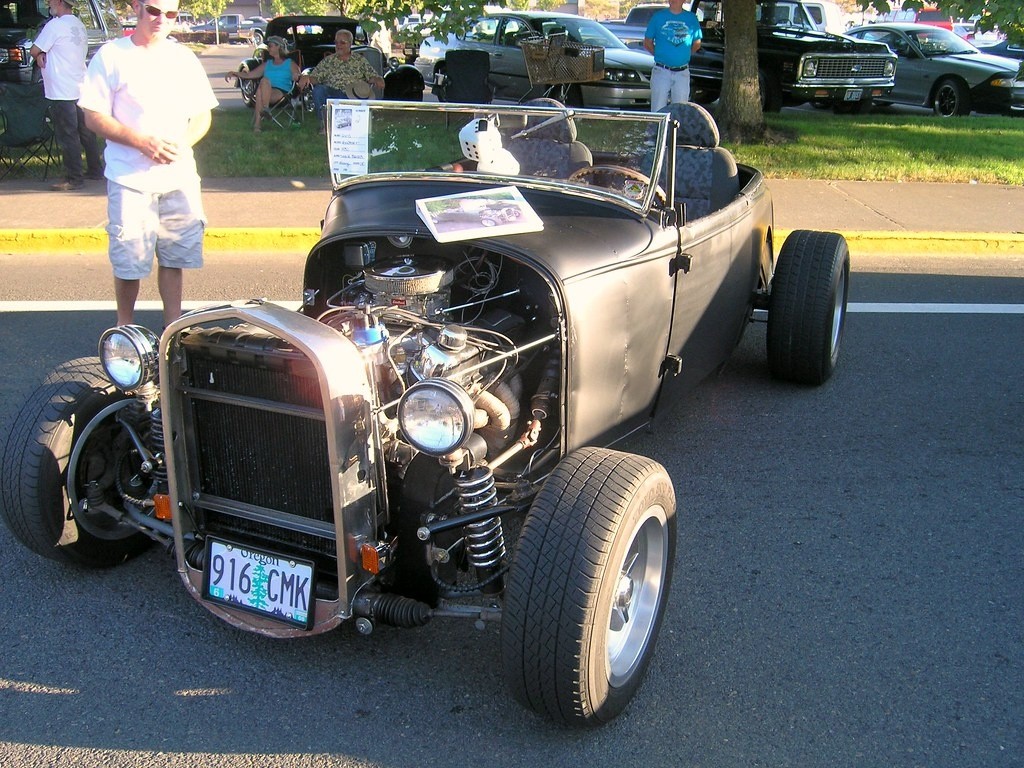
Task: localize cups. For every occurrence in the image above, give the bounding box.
[437,74,444,85]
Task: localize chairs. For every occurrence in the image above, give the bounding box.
[251,49,306,129]
[642,102,739,222]
[350,46,384,100]
[435,50,497,104]
[506,32,519,46]
[508,99,592,178]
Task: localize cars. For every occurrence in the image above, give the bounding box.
[843,22,1024,118]
[234,15,426,107]
[414,10,655,107]
[952,23,1005,48]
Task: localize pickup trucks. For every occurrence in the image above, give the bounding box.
[189,13,269,45]
[598,0,898,115]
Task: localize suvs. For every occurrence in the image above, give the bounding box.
[0,0,124,85]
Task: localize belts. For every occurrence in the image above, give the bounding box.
[656,62,687,71]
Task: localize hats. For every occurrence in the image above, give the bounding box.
[263,37,284,47]
[346,79,375,101]
[64,0,79,7]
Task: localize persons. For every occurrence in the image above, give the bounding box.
[644,0,702,146]
[225,36,301,132]
[299,30,385,134]
[30,0,104,190]
[78,0,219,330]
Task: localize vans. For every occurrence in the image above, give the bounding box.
[868,7,954,32]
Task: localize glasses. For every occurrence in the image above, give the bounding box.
[137,0,179,19]
[334,40,349,45]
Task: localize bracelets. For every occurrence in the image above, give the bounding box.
[41,52,46,54]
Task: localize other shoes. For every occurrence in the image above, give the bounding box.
[48,181,84,190]
[254,127,263,135]
[83,172,106,181]
[318,127,326,135]
[259,108,272,120]
